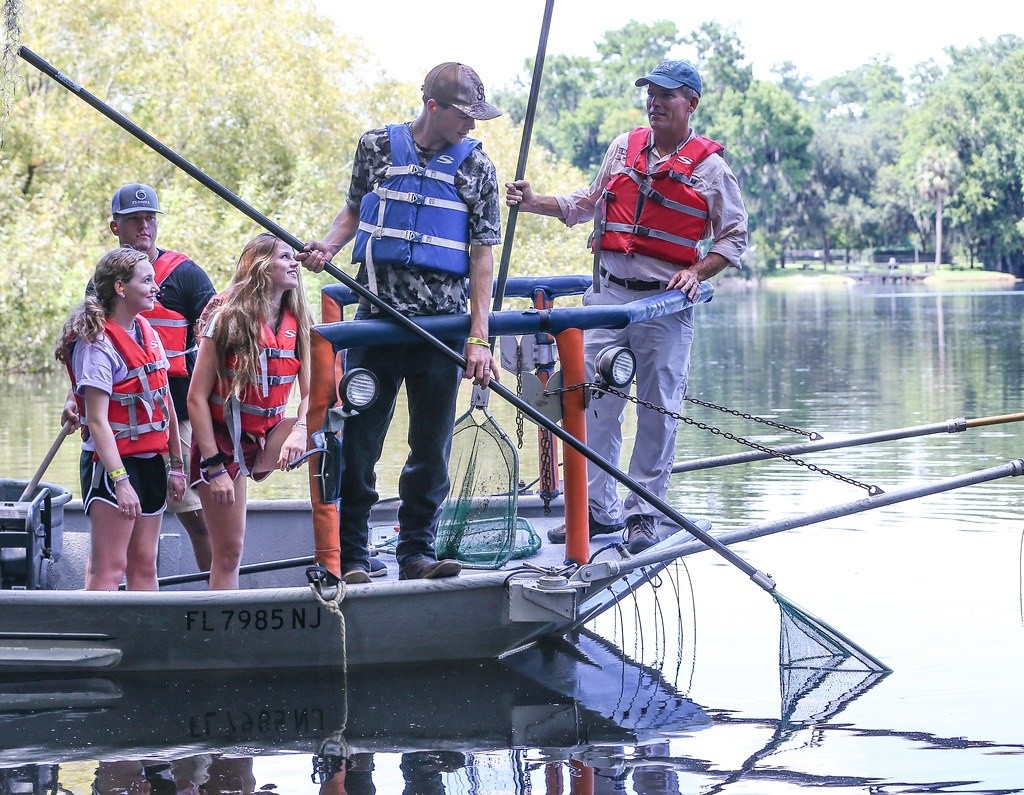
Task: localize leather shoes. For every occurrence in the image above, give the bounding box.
[341,566,372,584]
[399,559,462,579]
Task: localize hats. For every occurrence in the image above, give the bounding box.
[111,183,164,215]
[635,60,702,99]
[420,62,503,121]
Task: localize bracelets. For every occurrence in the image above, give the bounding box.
[208,470,227,480]
[109,467,127,478]
[114,474,130,488]
[201,452,226,469]
[295,421,308,429]
[169,471,188,480]
[169,459,184,468]
[465,337,492,349]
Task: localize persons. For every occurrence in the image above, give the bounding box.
[505,60,750,556]
[62,183,221,585]
[291,63,504,587]
[62,247,183,593]
[185,234,319,589]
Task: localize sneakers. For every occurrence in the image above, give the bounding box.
[622,514,660,555]
[367,556,387,576]
[547,506,625,544]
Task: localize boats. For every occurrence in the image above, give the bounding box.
[0,274,1024,672]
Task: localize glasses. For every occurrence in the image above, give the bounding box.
[631,144,678,180]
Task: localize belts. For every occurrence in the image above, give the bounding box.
[599,264,673,291]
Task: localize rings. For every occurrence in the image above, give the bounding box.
[484,368,491,371]
